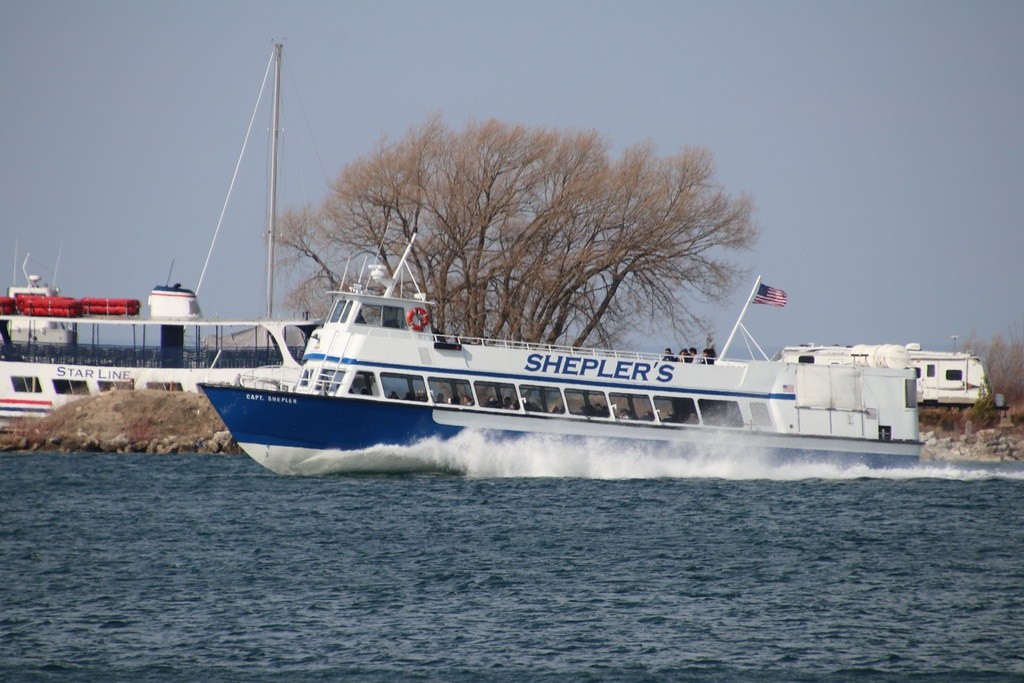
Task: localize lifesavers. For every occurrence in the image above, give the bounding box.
[407,307,430,331]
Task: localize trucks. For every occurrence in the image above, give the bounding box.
[782,342,993,406]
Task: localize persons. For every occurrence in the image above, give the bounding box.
[660,346,716,365]
[336,382,658,422]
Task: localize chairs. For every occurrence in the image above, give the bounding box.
[0,343,305,368]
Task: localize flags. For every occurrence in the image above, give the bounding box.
[751,283,788,307]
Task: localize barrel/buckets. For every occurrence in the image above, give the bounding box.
[996,395,1004,406]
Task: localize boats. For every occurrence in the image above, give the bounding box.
[195,233,920,476]
[0,252,324,418]
